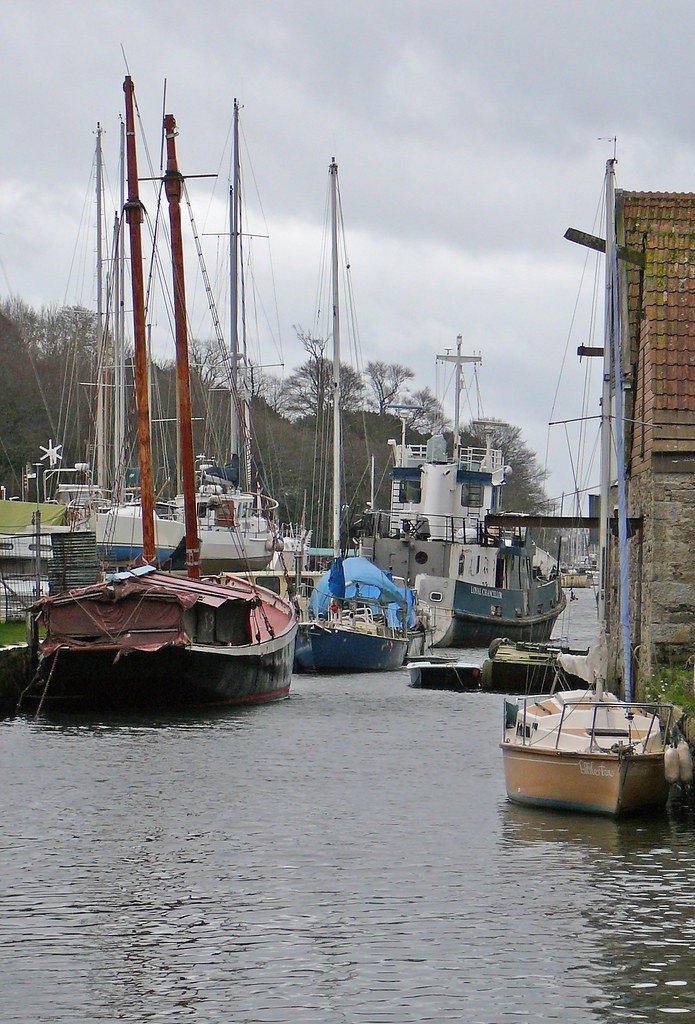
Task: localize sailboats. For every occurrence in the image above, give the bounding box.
[500,136,671,816]
[0,76,566,709]
